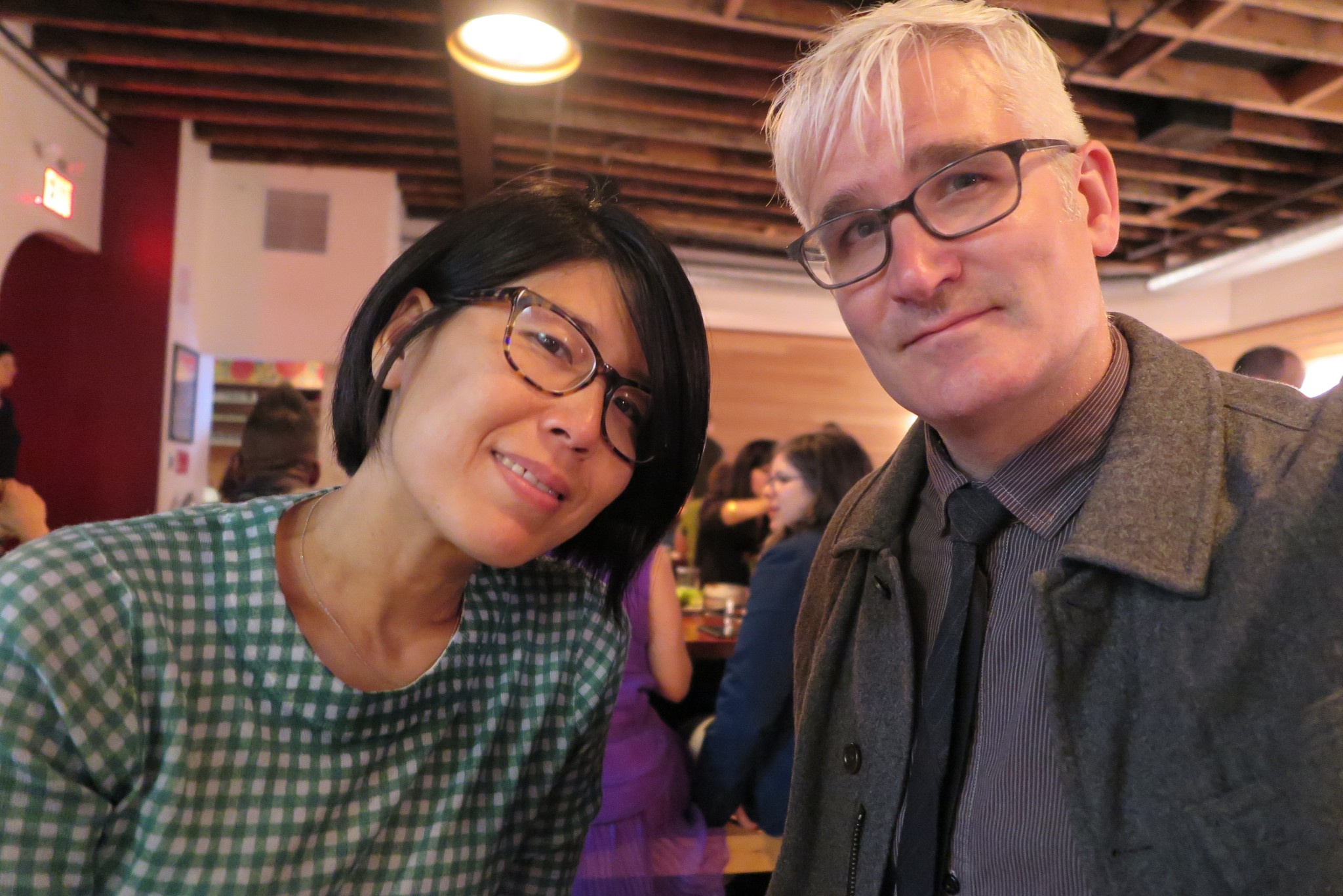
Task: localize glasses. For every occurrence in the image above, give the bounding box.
[758,467,802,492]
[435,287,662,465]
[782,139,1075,290]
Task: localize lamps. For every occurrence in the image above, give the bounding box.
[442,1,582,88]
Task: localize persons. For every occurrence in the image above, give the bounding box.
[761,1,1343,896]
[0,340,49,554]
[3,184,712,896]
[1233,345,1307,390]
[544,539,690,845]
[678,423,873,837]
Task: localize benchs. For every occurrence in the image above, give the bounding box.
[574,821,785,877]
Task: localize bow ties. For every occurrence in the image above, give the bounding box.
[898,485,1012,896]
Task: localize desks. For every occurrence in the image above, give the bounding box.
[680,610,746,660]
